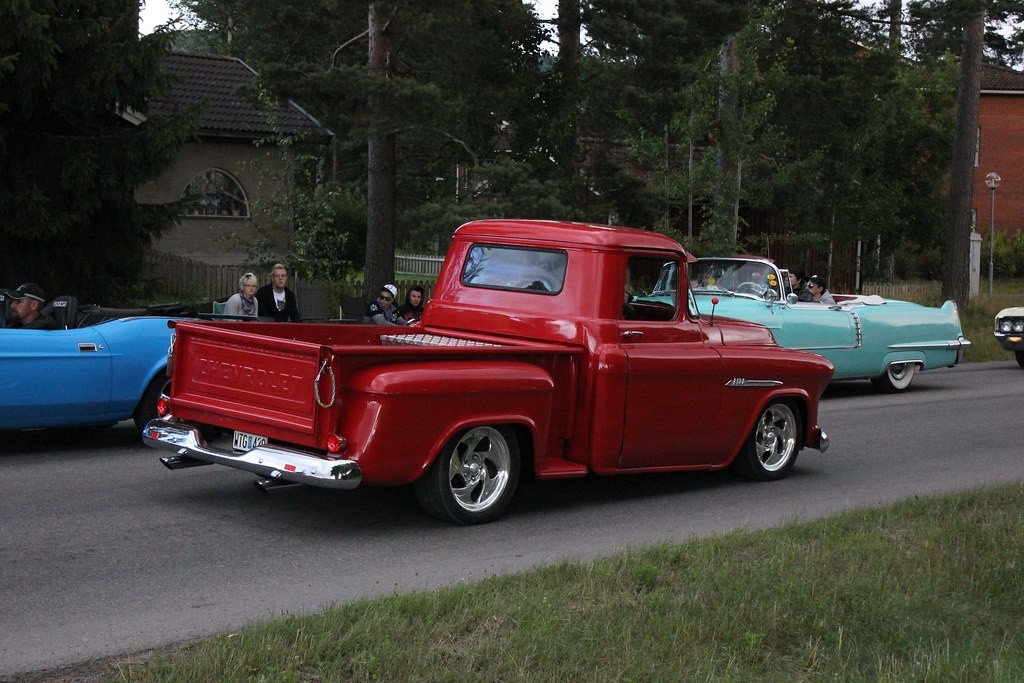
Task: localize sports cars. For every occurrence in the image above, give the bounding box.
[0,290,203,442]
[635,254,971,393]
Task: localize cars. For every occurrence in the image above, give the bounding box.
[992,304,1024,367]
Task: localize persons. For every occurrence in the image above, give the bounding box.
[362,283,408,326]
[223,272,259,322]
[749,257,838,306]
[689,262,727,292]
[5,282,59,330]
[398,284,426,328]
[255,262,301,323]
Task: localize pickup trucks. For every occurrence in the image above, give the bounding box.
[139,218,835,527]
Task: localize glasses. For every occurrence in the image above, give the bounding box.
[243,285,257,289]
[380,295,394,302]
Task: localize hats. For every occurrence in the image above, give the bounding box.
[4,283,46,303]
[384,284,398,298]
